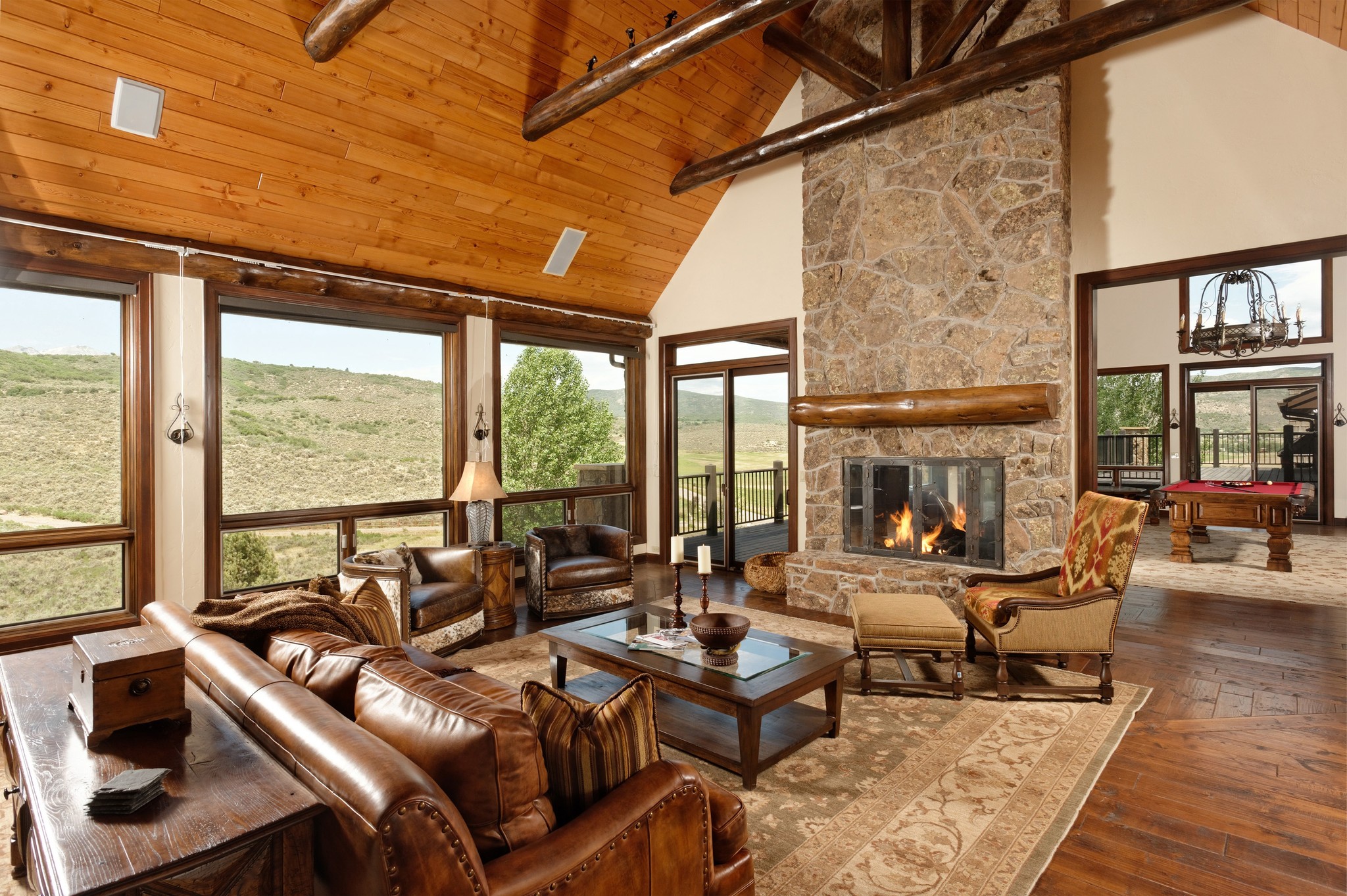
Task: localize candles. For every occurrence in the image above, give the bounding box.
[698,542,713,575]
[671,532,684,562]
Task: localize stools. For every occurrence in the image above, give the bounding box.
[850,595,964,697]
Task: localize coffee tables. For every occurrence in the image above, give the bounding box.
[539,604,856,789]
[453,540,516,629]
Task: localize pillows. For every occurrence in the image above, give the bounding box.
[263,576,659,858]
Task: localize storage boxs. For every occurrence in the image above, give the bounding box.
[65,624,186,746]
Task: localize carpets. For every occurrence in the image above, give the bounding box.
[453,593,1153,896]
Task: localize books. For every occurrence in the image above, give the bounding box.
[627,627,699,652]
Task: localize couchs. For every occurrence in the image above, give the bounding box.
[139,599,755,896]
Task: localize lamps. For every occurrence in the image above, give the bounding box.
[450,462,506,545]
[474,403,489,440]
[1332,402,1347,427]
[1177,267,1304,357]
[1168,408,1180,429]
[167,393,194,444]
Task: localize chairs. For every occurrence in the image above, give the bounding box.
[340,547,483,657]
[964,491,1148,702]
[527,524,633,617]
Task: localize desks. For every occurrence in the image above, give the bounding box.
[0,643,330,896]
[1164,477,1315,571]
